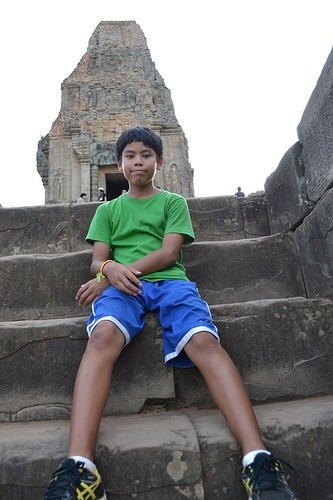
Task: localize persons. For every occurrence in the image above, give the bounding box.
[97,188,106,201]
[39,126,298,500]
[235,186,246,198]
[76,192,88,204]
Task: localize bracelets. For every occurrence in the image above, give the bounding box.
[96,260,114,283]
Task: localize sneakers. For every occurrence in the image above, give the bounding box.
[42,458,107,500]
[242,449,297,500]
